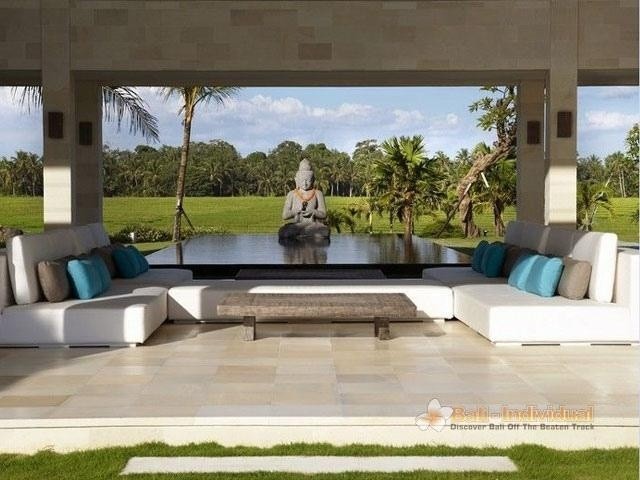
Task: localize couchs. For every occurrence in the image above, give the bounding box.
[422,220,640,347]
[0,222,193,350]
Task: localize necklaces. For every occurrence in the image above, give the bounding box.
[295,189,315,201]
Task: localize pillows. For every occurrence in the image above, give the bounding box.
[505,218,617,303]
[37,241,148,304]
[471,240,592,300]
[7,225,112,304]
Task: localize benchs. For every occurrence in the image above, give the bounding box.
[166,278,454,325]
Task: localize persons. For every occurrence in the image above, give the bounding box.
[279,158,331,240]
[278,240,331,264]
